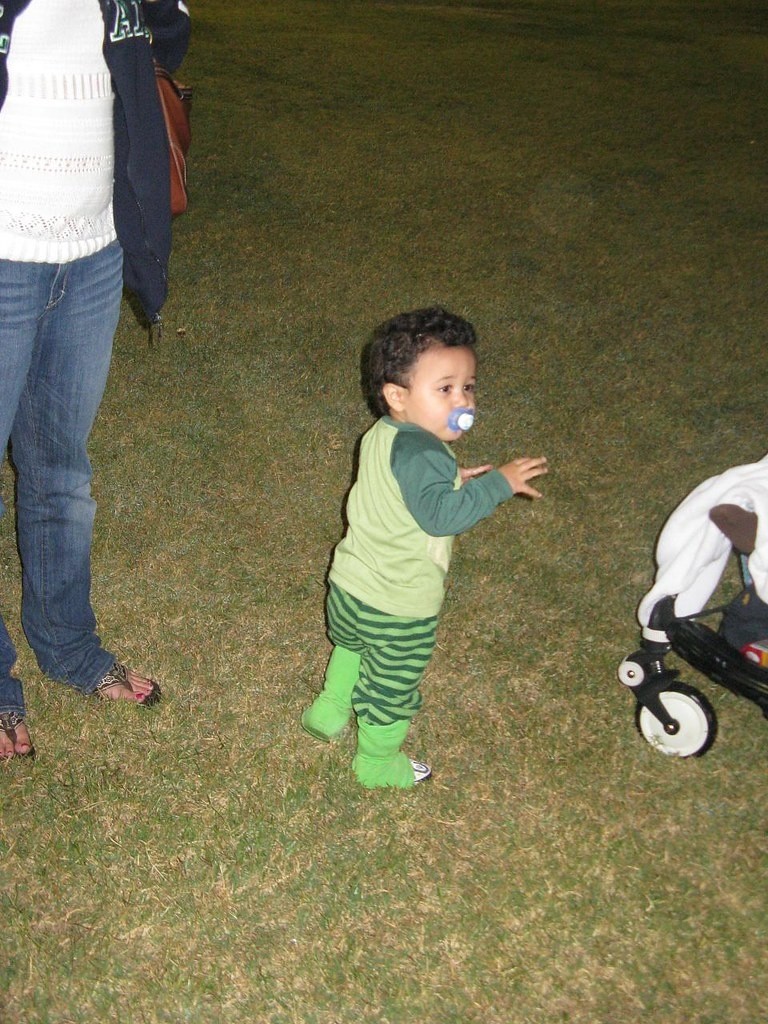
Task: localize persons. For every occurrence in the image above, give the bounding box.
[300,305,548,789]
[1,1,189,760]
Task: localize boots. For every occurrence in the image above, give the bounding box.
[351,716,432,789]
[302,646,360,739]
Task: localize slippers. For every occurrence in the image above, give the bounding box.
[1,712,35,763]
[93,663,162,709]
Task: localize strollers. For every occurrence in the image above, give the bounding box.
[615,447,768,766]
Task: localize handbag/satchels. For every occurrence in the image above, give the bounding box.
[152,66,192,221]
